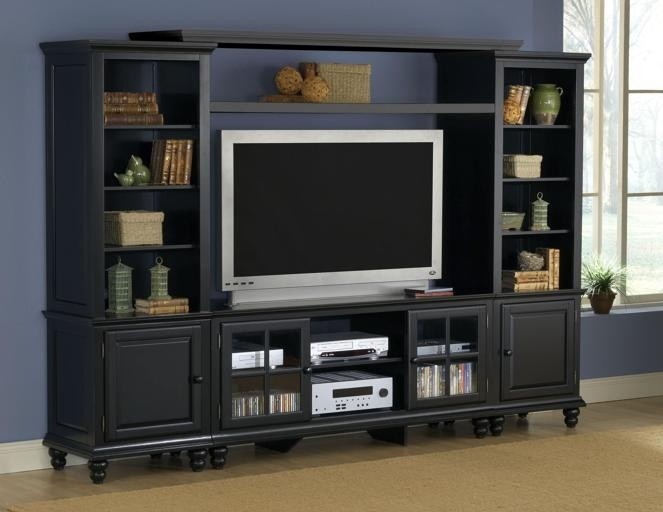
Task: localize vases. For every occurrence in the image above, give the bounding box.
[530,83,563,126]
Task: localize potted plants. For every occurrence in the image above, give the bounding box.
[581,255,634,314]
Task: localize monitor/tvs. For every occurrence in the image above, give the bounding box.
[215,129,443,305]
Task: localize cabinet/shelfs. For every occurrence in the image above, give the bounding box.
[40,29,592,482]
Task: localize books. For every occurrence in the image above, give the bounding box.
[150,139,193,185]
[450,361,477,395]
[135,297,190,314]
[502,247,560,292]
[417,364,445,398]
[270,391,301,415]
[505,84,532,125]
[104,91,164,125]
[232,391,264,418]
